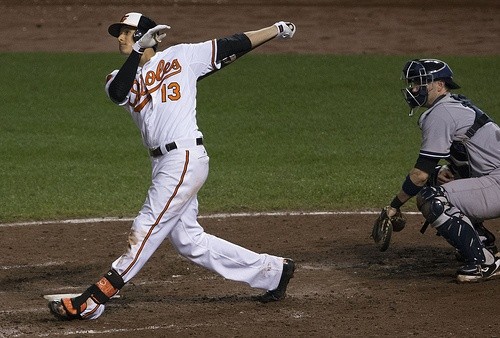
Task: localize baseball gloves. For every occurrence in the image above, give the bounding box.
[371,205,407,252]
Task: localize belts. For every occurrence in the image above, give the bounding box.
[150,137,203,158]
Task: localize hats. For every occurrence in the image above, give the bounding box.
[108,12,158,51]
[408,60,461,89]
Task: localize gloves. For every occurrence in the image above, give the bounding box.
[273,21,296,40]
[131,24,172,54]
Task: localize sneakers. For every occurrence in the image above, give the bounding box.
[49,300,75,319]
[456,246,500,282]
[260,258,295,302]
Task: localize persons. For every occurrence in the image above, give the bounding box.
[48,11,297,322]
[371,58,500,283]
